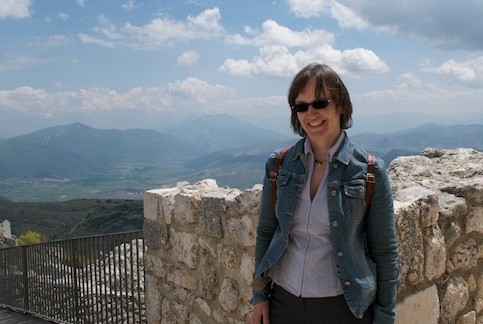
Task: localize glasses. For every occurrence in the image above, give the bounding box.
[291,96,335,114]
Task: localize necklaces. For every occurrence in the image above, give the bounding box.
[314,158,327,169]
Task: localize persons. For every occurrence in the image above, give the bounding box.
[251,62,400,324]
